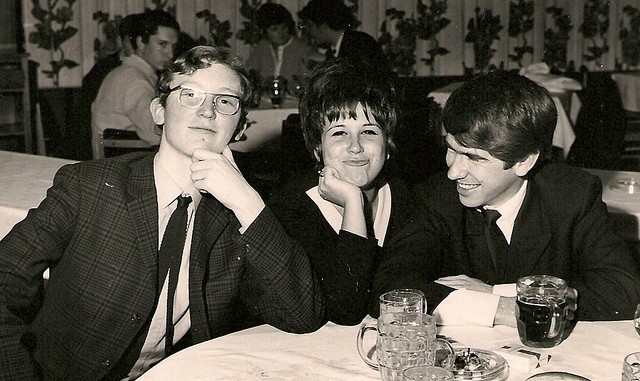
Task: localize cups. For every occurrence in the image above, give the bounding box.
[377,313,454,381]
[515,274,576,348]
[622,352,640,380]
[268,76,287,108]
[357,289,426,368]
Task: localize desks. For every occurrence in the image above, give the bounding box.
[0,149,104,232]
[133,311,640,380]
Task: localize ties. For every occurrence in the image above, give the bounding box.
[482,211,514,277]
[121,197,192,377]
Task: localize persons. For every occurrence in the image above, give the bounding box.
[0,46,325,381]
[372,69,640,330]
[79,12,143,154]
[298,0,393,93]
[264,57,419,325]
[90,9,181,161]
[254,2,312,99]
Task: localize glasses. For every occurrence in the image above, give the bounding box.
[166,85,242,116]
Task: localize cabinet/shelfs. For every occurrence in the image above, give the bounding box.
[1,50,34,150]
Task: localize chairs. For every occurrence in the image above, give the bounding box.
[98,132,152,156]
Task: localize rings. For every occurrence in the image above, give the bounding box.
[317,169,325,177]
